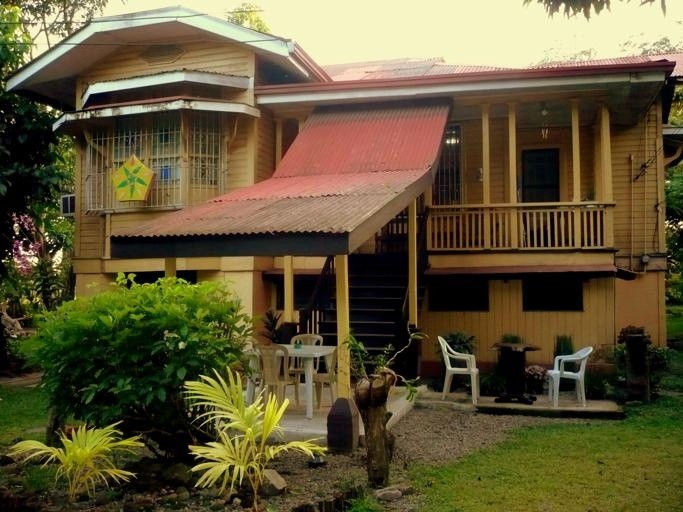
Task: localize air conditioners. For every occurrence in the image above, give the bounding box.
[60,194,75,216]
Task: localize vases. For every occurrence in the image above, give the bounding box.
[526,378,544,394]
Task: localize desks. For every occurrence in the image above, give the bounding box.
[241,344,337,419]
[491,341,544,404]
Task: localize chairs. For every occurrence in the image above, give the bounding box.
[253,333,338,416]
[544,344,593,408]
[437,335,481,407]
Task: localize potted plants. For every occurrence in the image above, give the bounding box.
[551,333,578,392]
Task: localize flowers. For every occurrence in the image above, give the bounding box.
[523,364,546,379]
